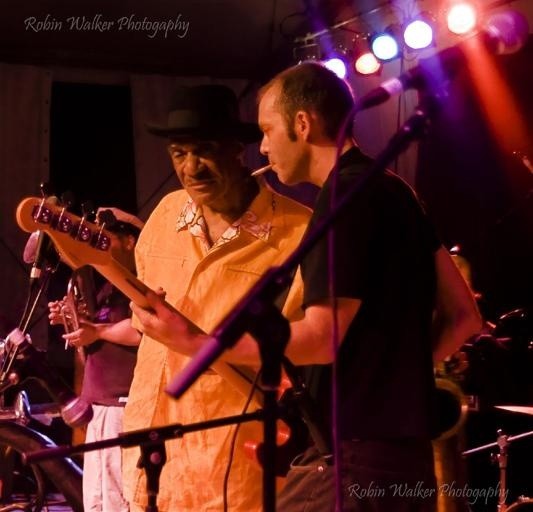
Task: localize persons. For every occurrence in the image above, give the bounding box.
[129,60,484,511]
[48,206,143,512]
[117,83,314,512]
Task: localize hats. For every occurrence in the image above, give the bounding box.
[95,207,145,234]
[139,82,256,142]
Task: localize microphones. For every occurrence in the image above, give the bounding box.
[282,356,334,466]
[29,196,60,289]
[360,44,467,110]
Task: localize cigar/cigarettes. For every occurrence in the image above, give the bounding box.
[250,164,272,176]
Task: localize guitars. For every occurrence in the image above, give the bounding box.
[438,307,524,379]
[15,183,468,481]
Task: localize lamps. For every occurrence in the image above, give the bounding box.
[294,3,475,79]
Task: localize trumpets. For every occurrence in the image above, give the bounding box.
[61,266,100,357]
